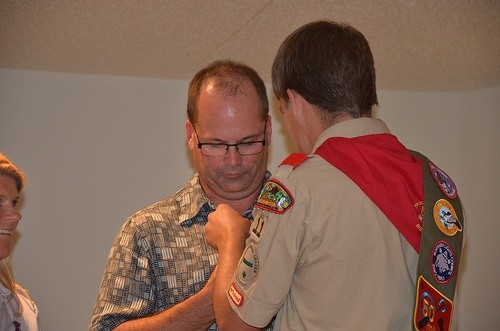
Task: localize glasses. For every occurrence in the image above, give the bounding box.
[190,120,266,155]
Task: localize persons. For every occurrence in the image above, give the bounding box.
[88,59,275,331]
[0,151,41,331]
[203,21,468,331]
